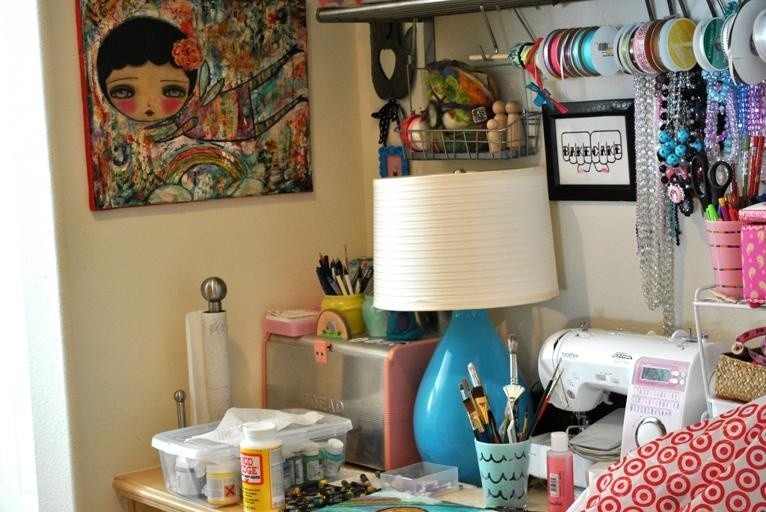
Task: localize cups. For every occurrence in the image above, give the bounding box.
[474,437,530,510]
[702,218,743,299]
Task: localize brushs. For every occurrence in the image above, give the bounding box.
[316,245,374,296]
[459,333,564,508]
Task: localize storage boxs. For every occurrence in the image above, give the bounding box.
[146,400,354,507]
[263,324,454,470]
[739,202,766,310]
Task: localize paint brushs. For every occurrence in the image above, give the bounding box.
[459,333,563,442]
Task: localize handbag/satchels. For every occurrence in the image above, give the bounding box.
[566,408,624,461]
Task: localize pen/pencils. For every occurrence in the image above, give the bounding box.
[707,136,765,221]
[317,244,372,294]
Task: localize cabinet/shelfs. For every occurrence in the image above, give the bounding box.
[692,284,764,443]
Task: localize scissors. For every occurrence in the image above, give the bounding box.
[692,156,732,217]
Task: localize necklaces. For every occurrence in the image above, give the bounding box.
[630,68,765,337]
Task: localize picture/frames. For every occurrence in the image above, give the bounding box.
[377,147,409,179]
[540,98,639,203]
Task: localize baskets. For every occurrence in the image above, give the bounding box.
[714,348,766,403]
[394,112,542,161]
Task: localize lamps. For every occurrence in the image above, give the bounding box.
[371,165,558,481]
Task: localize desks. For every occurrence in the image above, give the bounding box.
[115,419,766,511]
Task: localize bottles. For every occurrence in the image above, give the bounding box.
[291,440,343,485]
[204,464,238,506]
[173,456,202,497]
[238,421,287,512]
